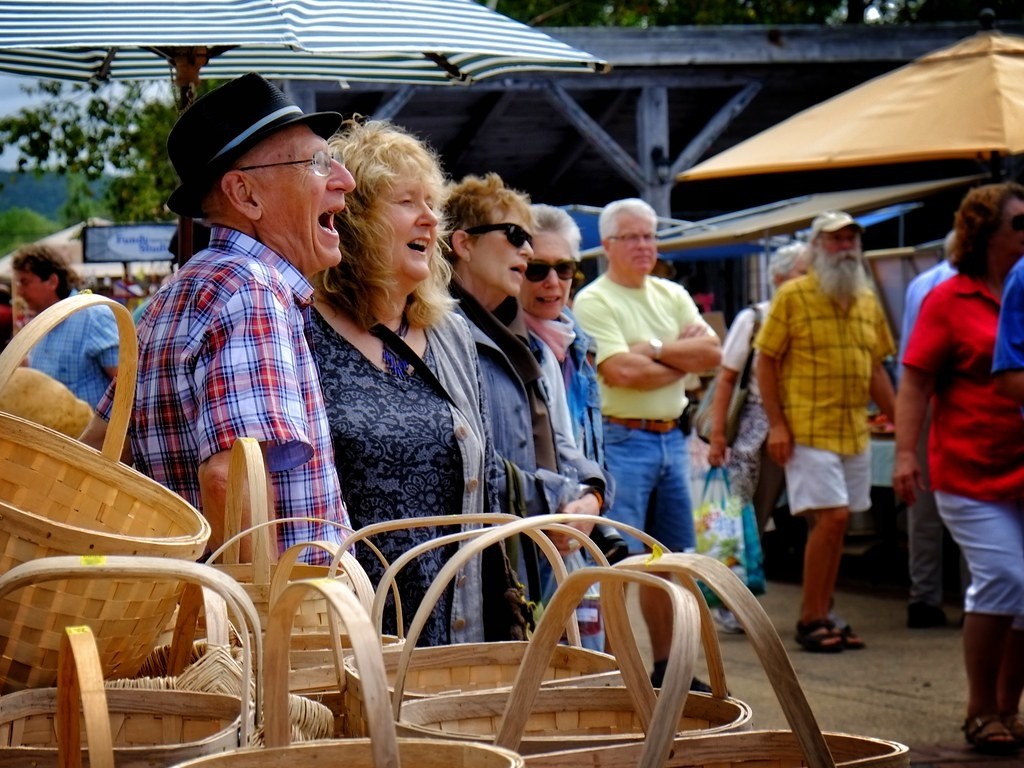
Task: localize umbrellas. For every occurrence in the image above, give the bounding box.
[0,0,612,270]
[675,8,1024,182]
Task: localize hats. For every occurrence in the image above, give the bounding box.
[808,210,865,241]
[165,70,344,218]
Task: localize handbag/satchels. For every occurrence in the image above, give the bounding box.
[692,373,750,448]
[691,464,767,608]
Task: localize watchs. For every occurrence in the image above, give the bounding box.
[649,339,663,359]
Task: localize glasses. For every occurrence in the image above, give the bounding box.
[605,231,656,242]
[465,222,534,249]
[524,260,579,282]
[1003,212,1024,233]
[235,150,337,178]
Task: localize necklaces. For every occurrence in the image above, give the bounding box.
[381,313,410,376]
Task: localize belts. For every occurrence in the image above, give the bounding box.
[608,417,681,433]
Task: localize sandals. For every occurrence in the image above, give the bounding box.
[794,619,844,653]
[1001,713,1024,750]
[961,713,1018,755]
[824,620,865,649]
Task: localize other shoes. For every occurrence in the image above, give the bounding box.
[649,672,731,698]
[910,602,947,627]
[713,608,746,634]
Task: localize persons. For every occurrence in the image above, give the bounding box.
[708,240,847,636]
[520,203,620,654]
[12,244,120,418]
[572,198,712,693]
[991,255,1024,402]
[306,120,535,649]
[442,172,608,607]
[895,231,971,630]
[77,71,356,565]
[892,180,1024,756]
[752,210,896,654]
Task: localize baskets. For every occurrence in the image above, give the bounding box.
[2,435,915,768]
[0,292,215,699]
